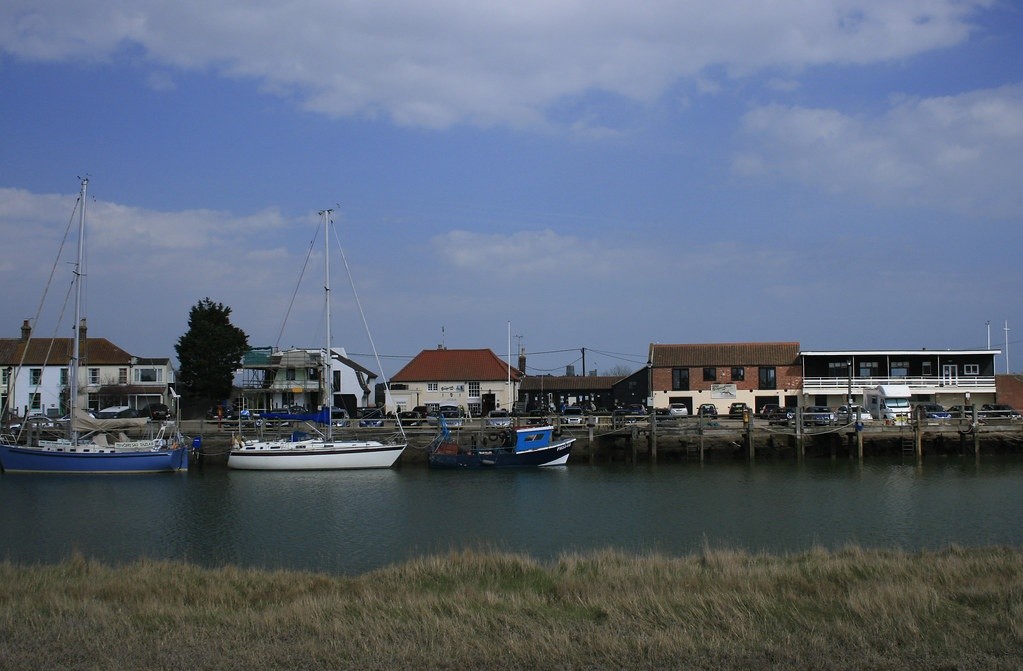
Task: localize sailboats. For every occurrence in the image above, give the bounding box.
[223,208,412,470]
[0,178,193,475]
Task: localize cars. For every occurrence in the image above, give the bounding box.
[767,407,798,424]
[696,403,718,419]
[802,405,839,425]
[759,404,782,419]
[330,407,350,428]
[946,405,984,420]
[412,406,432,423]
[208,406,234,421]
[625,404,647,415]
[561,406,585,424]
[612,409,637,424]
[58,410,97,424]
[395,411,424,425]
[360,408,385,426]
[728,402,749,418]
[981,403,1022,418]
[666,402,688,415]
[143,404,171,420]
[657,407,677,421]
[484,411,510,428]
[2,415,61,437]
[913,403,952,420]
[426,411,438,426]
[835,404,873,424]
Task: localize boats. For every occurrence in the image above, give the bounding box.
[425,411,578,468]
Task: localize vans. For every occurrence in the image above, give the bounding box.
[862,384,913,421]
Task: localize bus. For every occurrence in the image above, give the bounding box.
[438,403,464,431]
